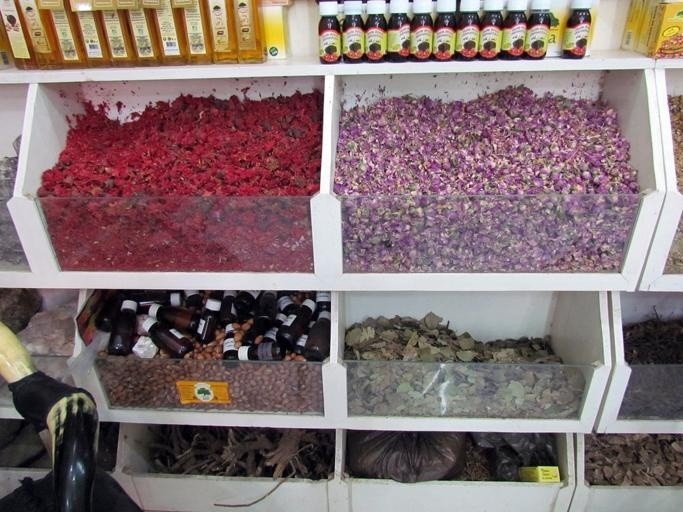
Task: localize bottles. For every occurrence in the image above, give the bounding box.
[0,0,290,71]
[317,0,553,65]
[93,290,330,366]
[560,0,593,59]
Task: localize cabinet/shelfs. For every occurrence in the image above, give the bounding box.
[1,47,683,510]
